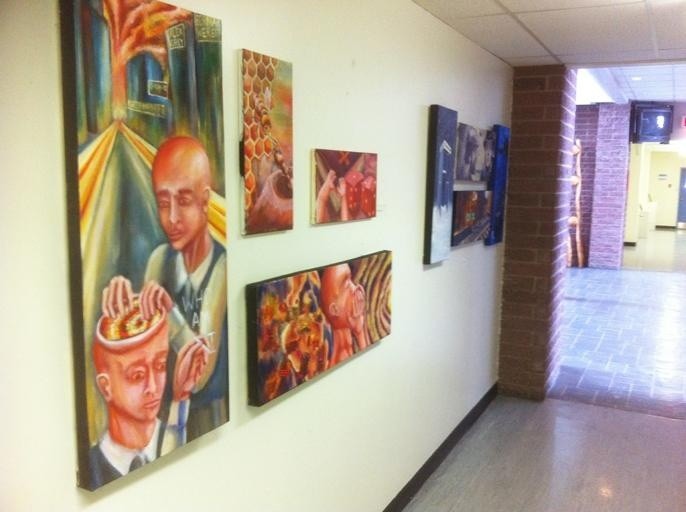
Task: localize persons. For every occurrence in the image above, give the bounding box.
[316,169,349,223]
[315,263,372,370]
[97,133,227,443]
[90,294,212,489]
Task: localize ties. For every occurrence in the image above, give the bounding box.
[633,104,673,144]
